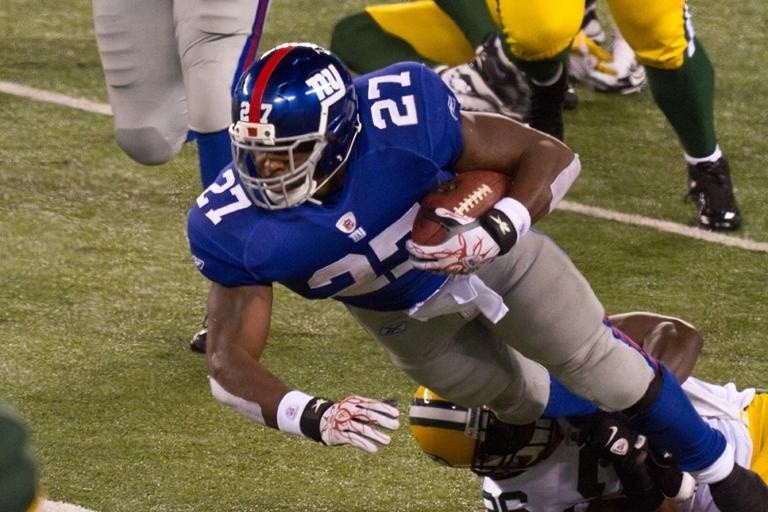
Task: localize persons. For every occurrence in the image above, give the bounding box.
[0,409,88,510]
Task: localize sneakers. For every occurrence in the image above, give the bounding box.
[190,329,208,353]
[685,157,743,231]
[522,77,570,142]
[604,26,648,96]
[640,448,704,501]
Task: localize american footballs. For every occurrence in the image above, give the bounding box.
[412,170,512,250]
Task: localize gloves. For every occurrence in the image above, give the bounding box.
[597,422,653,469]
[402,202,526,281]
[317,392,404,453]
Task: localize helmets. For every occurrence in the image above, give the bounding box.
[231,41,364,181]
[407,383,554,474]
